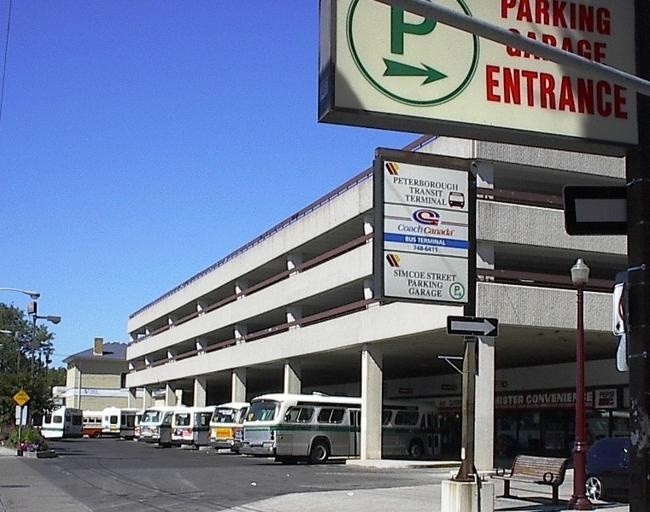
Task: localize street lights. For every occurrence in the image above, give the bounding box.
[569,258,597,511]
[0,329,13,337]
[0,287,41,300]
[26,314,63,436]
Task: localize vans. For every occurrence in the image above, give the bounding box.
[582,435,632,501]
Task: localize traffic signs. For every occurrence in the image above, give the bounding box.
[0,343,7,349]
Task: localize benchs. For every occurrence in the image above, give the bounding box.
[487,452,573,507]
